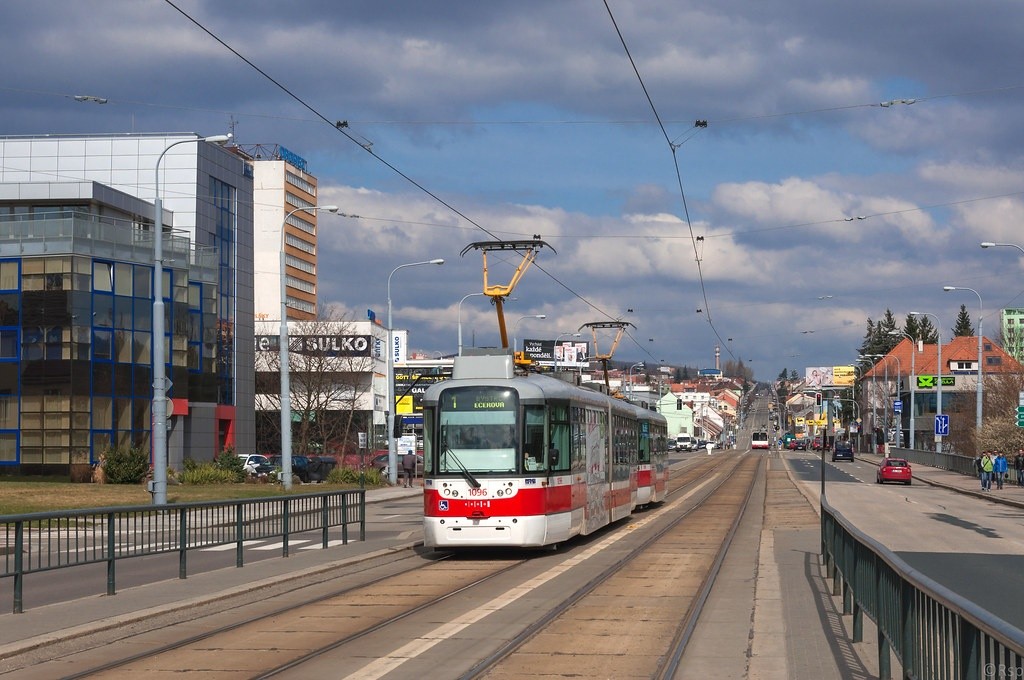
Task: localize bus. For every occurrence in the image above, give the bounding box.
[420,233,669,559]
[751,429,770,449]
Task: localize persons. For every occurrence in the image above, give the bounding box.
[464,427,479,449]
[502,427,529,459]
[402,450,415,487]
[1014,450,1024,488]
[706,443,712,455]
[727,443,730,449]
[976,449,998,492]
[993,451,1008,490]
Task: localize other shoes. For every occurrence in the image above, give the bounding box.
[982,487,984,490]
[997,485,999,489]
[988,489,990,492]
[1000,486,1002,489]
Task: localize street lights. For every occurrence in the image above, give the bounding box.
[888,331,915,450]
[877,354,900,449]
[512,315,547,353]
[909,312,943,453]
[458,292,485,357]
[385,258,447,486]
[276,206,341,491]
[659,385,664,414]
[848,354,889,460]
[943,286,984,459]
[629,363,645,401]
[147,133,231,515]
[553,333,581,372]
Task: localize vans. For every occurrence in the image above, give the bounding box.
[674,433,693,452]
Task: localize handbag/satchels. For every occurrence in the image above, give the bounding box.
[980,467,984,473]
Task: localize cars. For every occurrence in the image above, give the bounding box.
[831,440,855,463]
[756,393,765,399]
[767,392,780,431]
[667,439,677,452]
[751,408,756,412]
[690,435,736,451]
[777,433,831,453]
[368,453,424,478]
[876,456,912,485]
[236,452,336,489]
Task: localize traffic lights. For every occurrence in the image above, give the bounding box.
[816,393,823,406]
